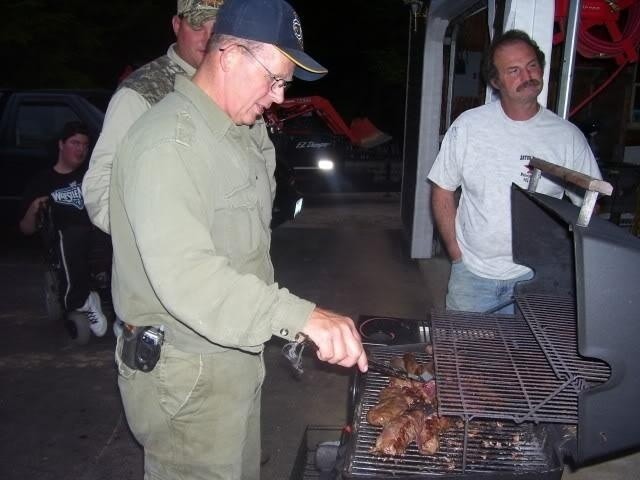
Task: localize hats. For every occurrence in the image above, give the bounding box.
[177,0,225,25]
[212,0,329,82]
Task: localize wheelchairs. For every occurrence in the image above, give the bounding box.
[34,199,91,346]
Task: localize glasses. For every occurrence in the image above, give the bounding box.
[219,44,287,92]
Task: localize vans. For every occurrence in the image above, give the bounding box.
[1,92,107,211]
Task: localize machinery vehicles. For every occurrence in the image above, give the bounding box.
[261,96,393,182]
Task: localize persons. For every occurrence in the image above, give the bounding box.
[109,0,368,480]
[17,121,123,339]
[426,29,607,313]
[81,0,224,236]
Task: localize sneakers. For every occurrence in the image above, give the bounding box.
[77,291,108,337]
